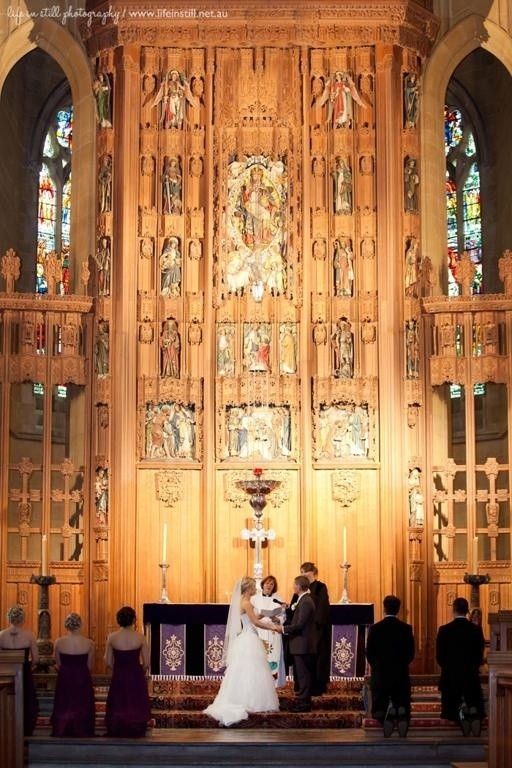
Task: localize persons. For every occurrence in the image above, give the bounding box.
[406,72,419,128]
[330,71,356,378]
[405,238,418,297]
[403,155,421,213]
[235,164,278,252]
[435,596,486,738]
[93,469,109,526]
[364,595,416,739]
[415,488,425,525]
[158,69,185,379]
[204,565,332,721]
[217,321,296,376]
[52,611,98,738]
[140,402,371,460]
[103,605,152,738]
[95,321,109,379]
[1,604,42,737]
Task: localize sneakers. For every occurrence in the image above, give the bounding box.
[289,703,311,712]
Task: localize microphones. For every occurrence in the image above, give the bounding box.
[272,598,288,607]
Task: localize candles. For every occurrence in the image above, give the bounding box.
[41,534,48,577]
[472,536,478,576]
[161,525,166,566]
[343,527,347,563]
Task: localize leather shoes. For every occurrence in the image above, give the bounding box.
[458,702,471,736]
[397,699,411,738]
[471,702,483,738]
[383,698,397,738]
